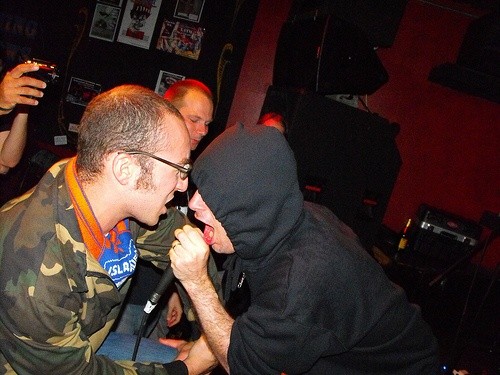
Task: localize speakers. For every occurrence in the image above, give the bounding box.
[258,90,402,225]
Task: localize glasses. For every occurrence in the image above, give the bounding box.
[118,150,193,181]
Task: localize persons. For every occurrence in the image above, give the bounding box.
[256,112,287,135]
[0,64,46,174]
[0,78,225,375]
[170,120,440,375]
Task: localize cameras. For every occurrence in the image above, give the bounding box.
[23,57,62,90]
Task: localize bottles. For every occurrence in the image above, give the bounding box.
[355,200,377,247]
[303,185,321,203]
[395,218,413,257]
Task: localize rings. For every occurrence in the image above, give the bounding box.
[173,243,181,250]
